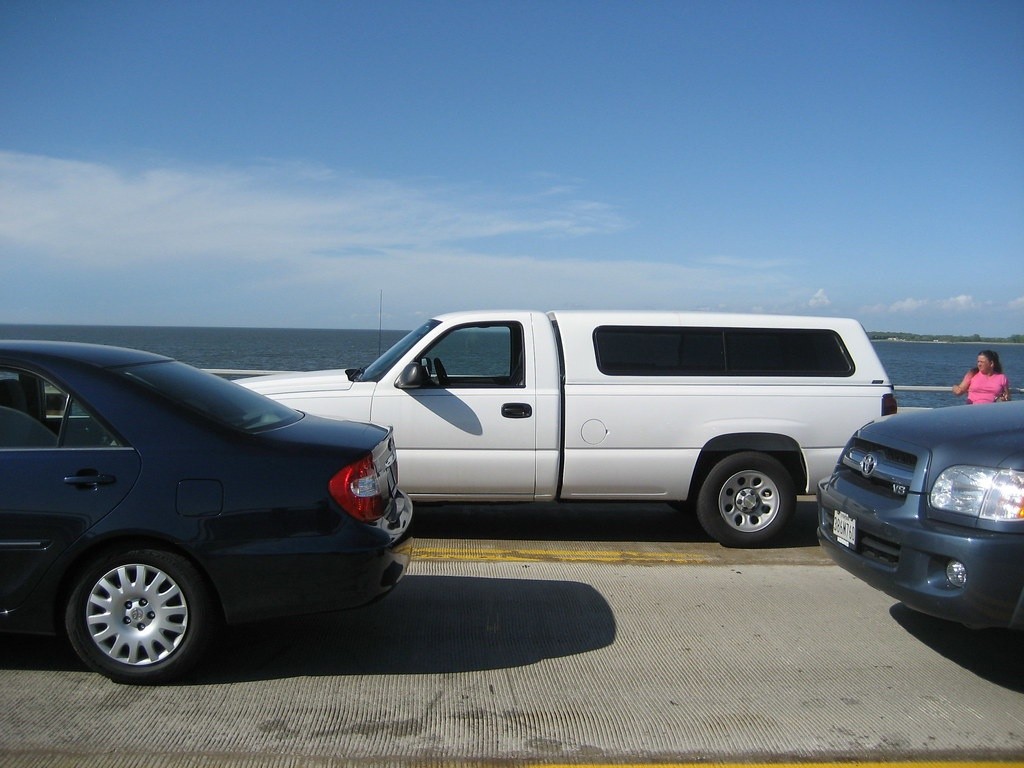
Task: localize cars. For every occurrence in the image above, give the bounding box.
[815,399,1023,635]
[0,337,415,689]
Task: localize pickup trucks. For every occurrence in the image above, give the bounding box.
[228,309,898,548]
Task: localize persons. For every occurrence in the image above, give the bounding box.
[952,350,1010,405]
